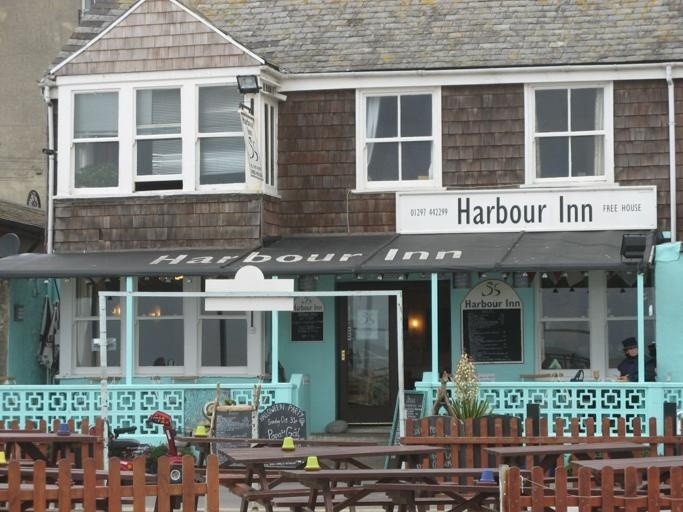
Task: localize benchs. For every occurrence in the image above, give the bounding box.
[0,465,208,508]
[278,463,541,511]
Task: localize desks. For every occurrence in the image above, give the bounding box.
[172,435,448,512]
[482,441,682,512]
[0,429,98,508]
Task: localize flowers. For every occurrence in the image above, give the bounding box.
[447,352,495,420]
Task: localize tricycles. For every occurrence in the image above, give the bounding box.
[1,409,184,510]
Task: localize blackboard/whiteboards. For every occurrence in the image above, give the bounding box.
[460,279,524,364]
[404,391,427,420]
[210,406,257,464]
[258,403,306,439]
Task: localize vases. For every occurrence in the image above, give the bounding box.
[410,413,520,485]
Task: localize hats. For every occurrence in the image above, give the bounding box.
[621,337,638,350]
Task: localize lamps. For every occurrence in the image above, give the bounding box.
[616,231,645,276]
[233,73,259,114]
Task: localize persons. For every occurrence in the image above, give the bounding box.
[617,337,657,382]
